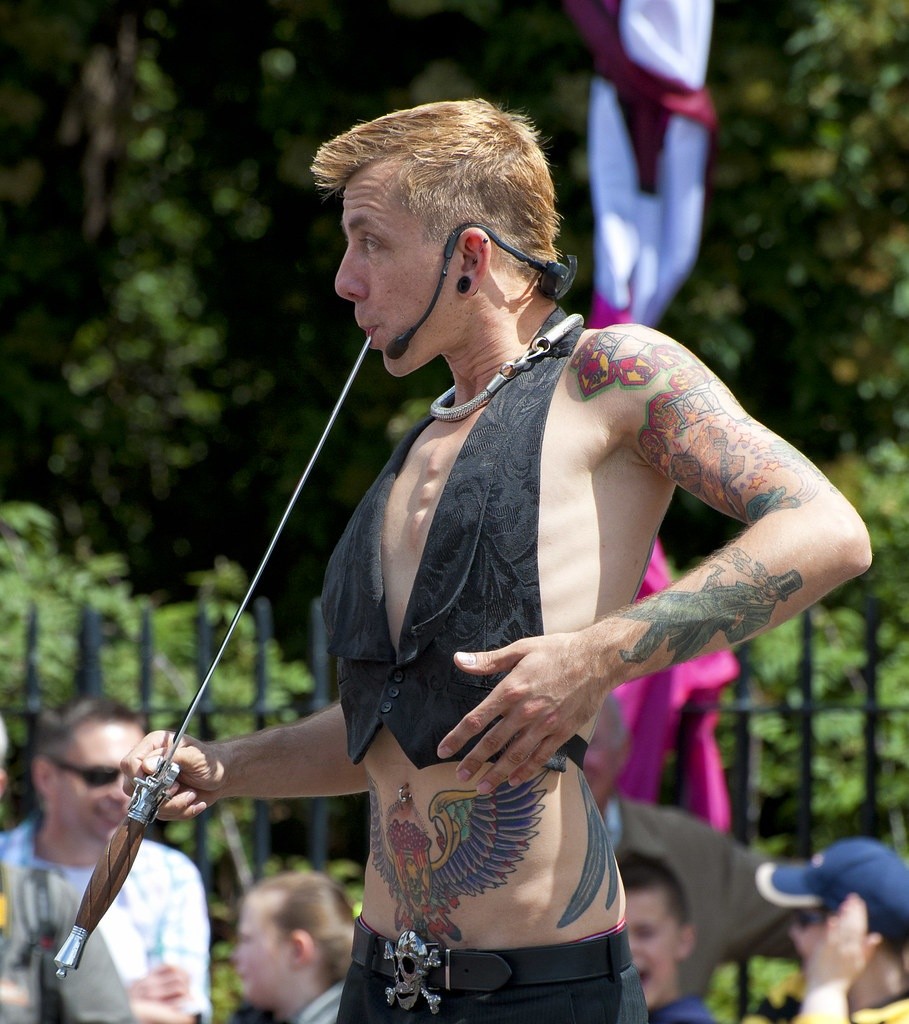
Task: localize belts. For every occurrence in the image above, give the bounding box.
[350,911,632,1015]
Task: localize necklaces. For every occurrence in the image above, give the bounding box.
[430,314,585,422]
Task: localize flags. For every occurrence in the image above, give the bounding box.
[571,0,739,835]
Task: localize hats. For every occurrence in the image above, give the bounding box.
[756,838,909,952]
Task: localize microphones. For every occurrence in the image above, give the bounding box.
[385,233,460,360]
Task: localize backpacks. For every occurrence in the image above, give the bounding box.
[0,868,59,1021]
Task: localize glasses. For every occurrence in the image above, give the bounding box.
[42,751,122,784]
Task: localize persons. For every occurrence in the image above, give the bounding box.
[583,690,792,998]
[741,836,909,1023]
[0,714,137,1024]
[614,853,715,1024]
[121,100,872,1024]
[0,691,213,1024]
[232,871,355,1024]
